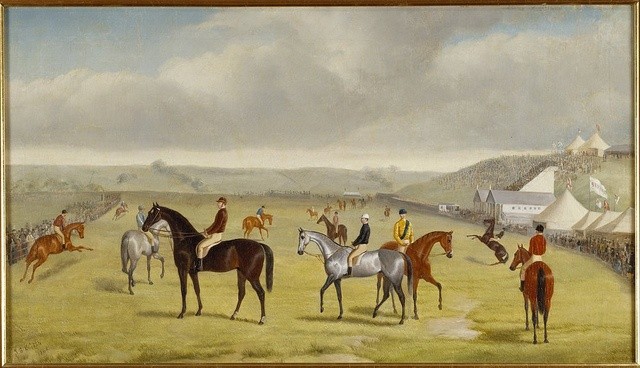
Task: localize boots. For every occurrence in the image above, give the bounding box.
[192,258,201,270]
[343,267,352,276]
[519,280,524,291]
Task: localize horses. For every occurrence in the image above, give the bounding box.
[351,200,356,208]
[376,230,454,320]
[317,213,348,247]
[142,202,274,325]
[509,243,554,344]
[384,207,392,222]
[111,204,128,222]
[297,226,414,325]
[343,201,346,211]
[324,206,332,215]
[242,214,273,241]
[306,208,318,220]
[467,218,509,266]
[337,199,343,210]
[19,221,94,284]
[120,219,172,295]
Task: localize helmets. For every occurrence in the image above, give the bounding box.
[536,224,544,232]
[216,196,226,202]
[399,208,407,214]
[360,213,369,218]
[62,209,67,214]
